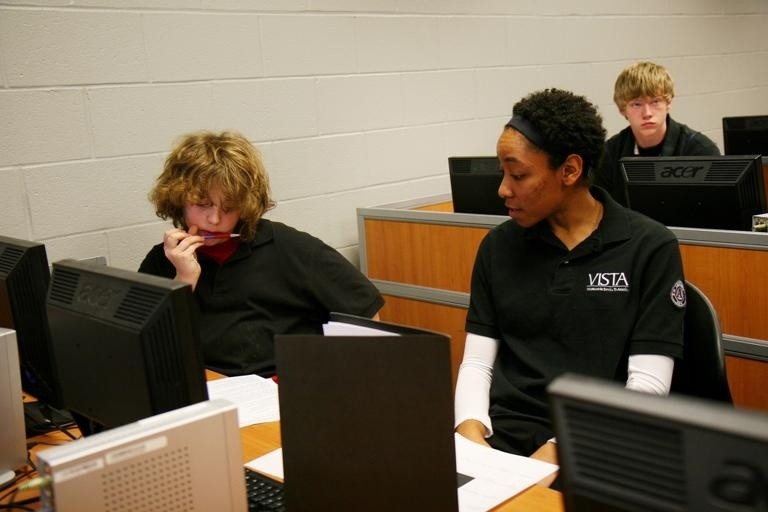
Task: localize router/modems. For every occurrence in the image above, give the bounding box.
[37,399,249,512]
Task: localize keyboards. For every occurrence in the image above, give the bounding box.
[242,466,285,512]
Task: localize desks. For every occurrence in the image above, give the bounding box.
[34,362,571,512]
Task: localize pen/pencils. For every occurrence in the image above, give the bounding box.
[203,231,239,242]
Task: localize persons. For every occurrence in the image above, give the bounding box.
[454,83,691,466]
[137,129,387,377]
[604,60,722,157]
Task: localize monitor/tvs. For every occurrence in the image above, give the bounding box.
[44,259,209,437]
[546,372,768,512]
[0,236,77,438]
[619,155,767,231]
[448,156,509,215]
[723,116,768,157]
